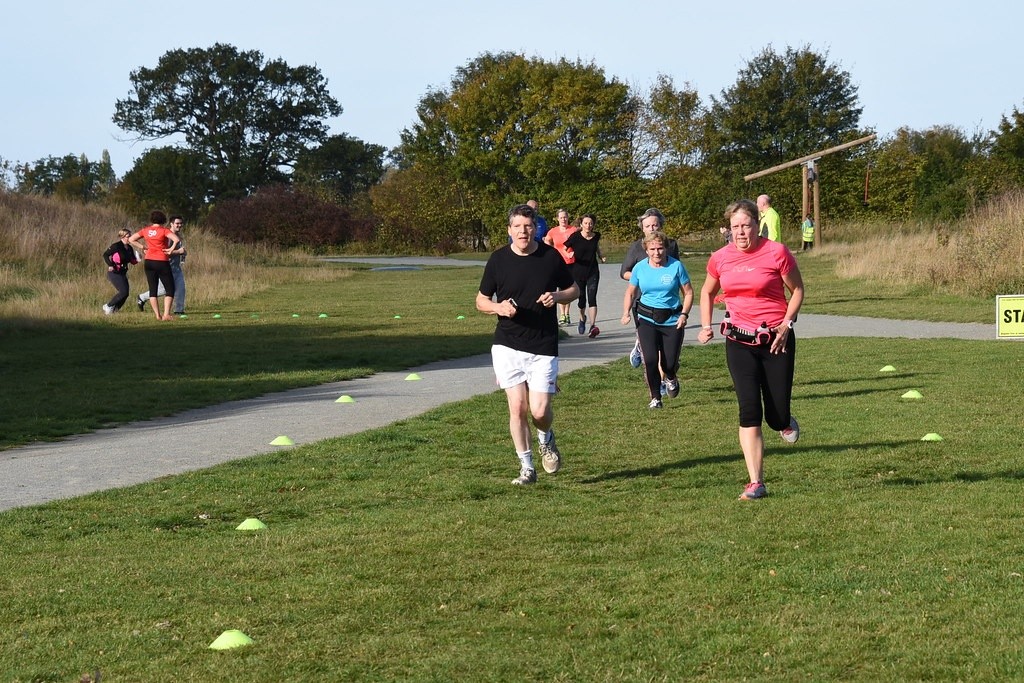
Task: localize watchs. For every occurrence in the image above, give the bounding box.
[783,320,793,328]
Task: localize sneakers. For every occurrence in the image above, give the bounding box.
[665,375,679,398]
[538,430,560,473]
[660,380,667,395]
[780,416,799,443]
[630,340,641,368]
[649,397,662,409]
[737,482,768,501]
[511,467,536,485]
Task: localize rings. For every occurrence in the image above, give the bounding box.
[780,344,782,345]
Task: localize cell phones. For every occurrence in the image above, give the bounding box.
[509,299,517,309]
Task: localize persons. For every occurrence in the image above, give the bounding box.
[757,195,781,243]
[102,229,141,313]
[128,211,180,321]
[476,204,580,484]
[137,216,187,314]
[698,199,804,500]
[563,214,606,338]
[620,208,680,395]
[621,231,694,408]
[719,223,733,243]
[801,213,814,250]
[544,208,578,325]
[510,200,546,243]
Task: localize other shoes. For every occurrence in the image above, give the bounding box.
[103,304,115,316]
[137,294,145,311]
[588,325,600,338]
[559,316,566,324]
[566,314,570,324]
[578,315,587,334]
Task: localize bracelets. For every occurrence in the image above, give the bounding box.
[681,313,688,319]
[703,326,710,328]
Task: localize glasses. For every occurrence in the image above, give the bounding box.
[174,222,182,225]
